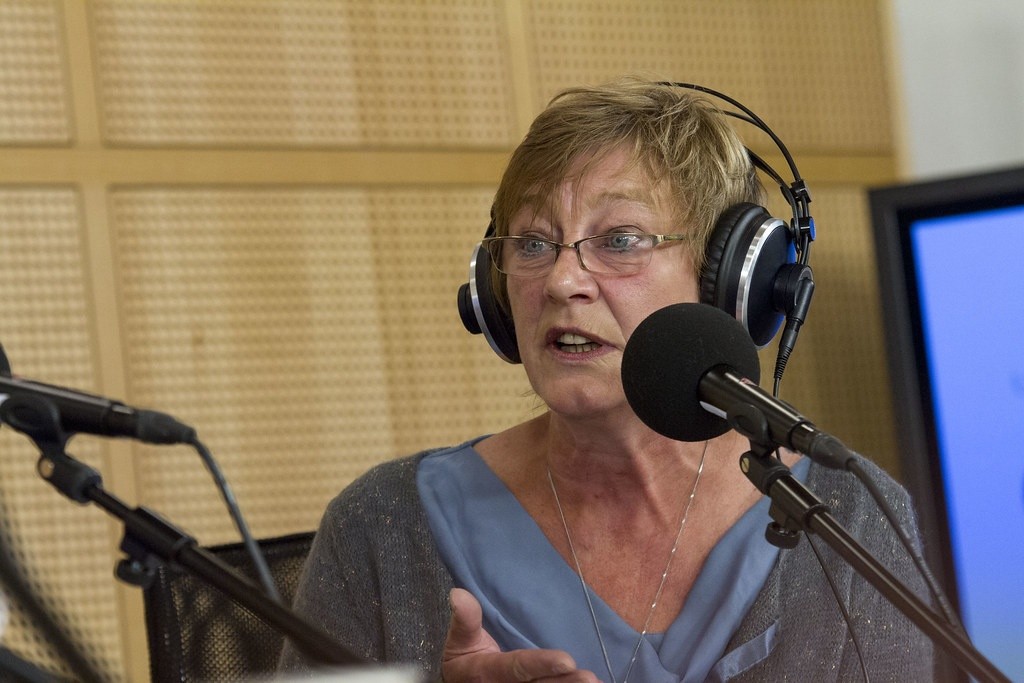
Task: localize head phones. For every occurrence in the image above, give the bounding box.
[457,82,815,378]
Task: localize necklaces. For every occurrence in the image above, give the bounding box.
[546,440,709,683]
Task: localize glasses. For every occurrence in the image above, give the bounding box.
[482,232,697,278]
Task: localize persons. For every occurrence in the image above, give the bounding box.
[278,85,935,683]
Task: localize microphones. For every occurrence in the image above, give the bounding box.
[0,343,196,446]
[621,303,857,472]
